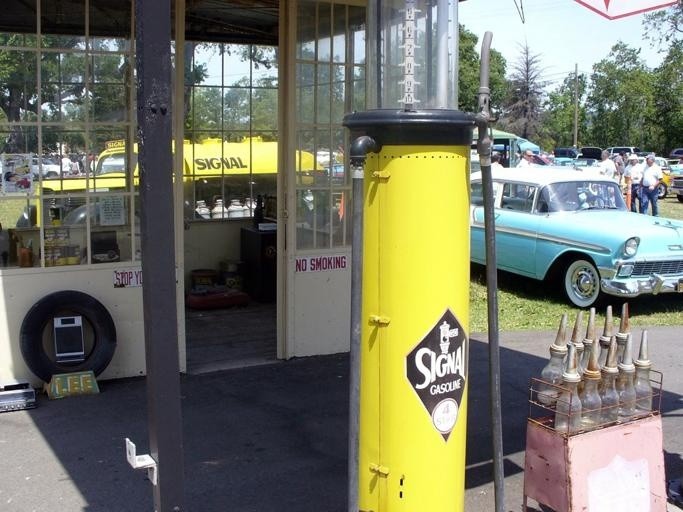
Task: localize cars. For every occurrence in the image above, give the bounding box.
[470,165,683,314]
[33,158,60,178]
[548,147,683,202]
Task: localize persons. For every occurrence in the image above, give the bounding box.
[598,150,663,217]
[679,157,683,164]
[303,143,344,165]
[490,153,504,170]
[44,148,92,177]
[514,149,555,167]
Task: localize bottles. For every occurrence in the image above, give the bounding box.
[537,303,653,432]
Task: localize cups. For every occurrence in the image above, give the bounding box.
[19,248,35,267]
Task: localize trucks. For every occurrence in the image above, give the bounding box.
[16,136,325,266]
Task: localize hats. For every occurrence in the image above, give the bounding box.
[628,154,639,160]
[645,155,655,162]
[526,150,536,156]
